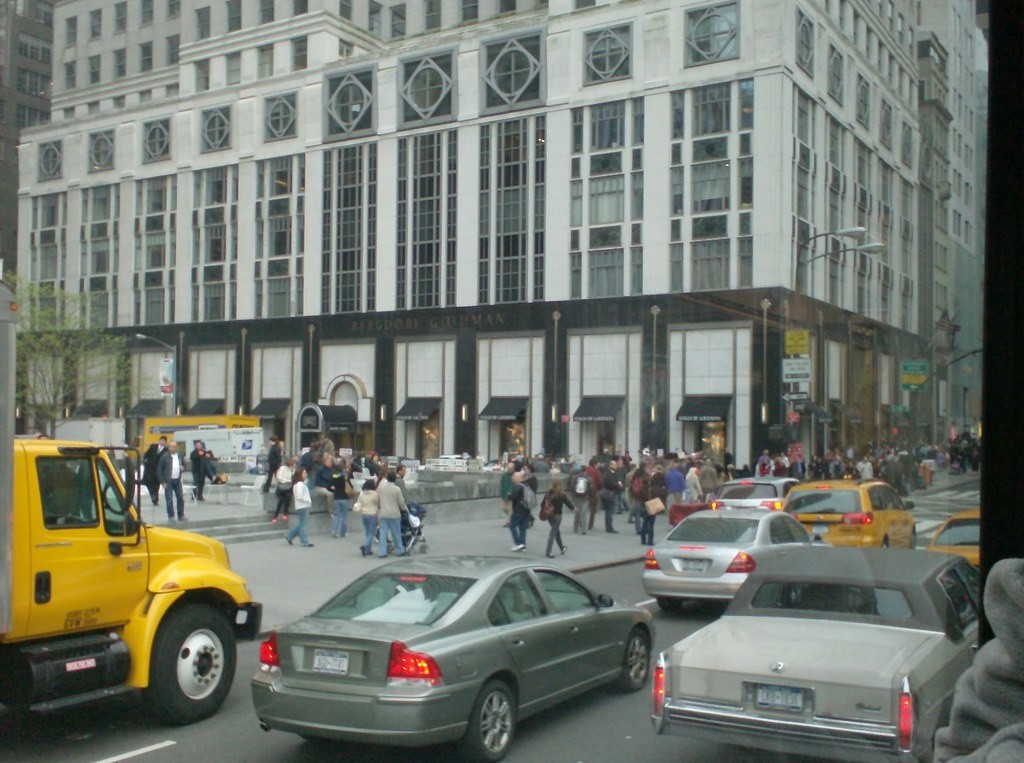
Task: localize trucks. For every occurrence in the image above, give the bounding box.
[1,435,263,727]
[128,415,263,465]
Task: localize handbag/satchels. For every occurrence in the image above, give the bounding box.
[575,477,588,494]
[408,514,420,528]
[599,486,615,503]
[644,497,665,516]
[527,514,535,528]
[279,483,293,490]
[539,499,555,521]
[344,473,355,498]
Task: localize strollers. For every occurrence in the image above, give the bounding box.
[377,502,429,556]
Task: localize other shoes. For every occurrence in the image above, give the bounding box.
[284,536,293,545]
[272,518,277,523]
[332,530,339,538]
[360,545,366,557]
[178,516,188,521]
[520,549,526,552]
[561,545,567,554]
[345,532,349,537]
[546,552,555,557]
[330,512,336,519]
[367,552,373,555]
[304,543,314,547]
[282,511,287,519]
[606,529,618,533]
[511,544,524,551]
[169,517,176,522]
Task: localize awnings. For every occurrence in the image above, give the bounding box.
[882,404,928,427]
[71,400,105,418]
[301,403,356,431]
[125,398,164,419]
[571,395,626,422]
[478,395,528,421]
[187,398,224,415]
[676,393,733,421]
[396,397,440,421]
[806,400,862,423]
[251,397,290,419]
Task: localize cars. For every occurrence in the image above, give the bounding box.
[643,504,825,610]
[648,545,978,763]
[928,511,981,568]
[713,477,800,511]
[250,553,656,763]
[782,481,918,550]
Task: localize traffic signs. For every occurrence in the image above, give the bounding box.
[782,392,809,402]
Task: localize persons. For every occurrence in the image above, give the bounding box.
[142,435,221,524]
[261,432,411,559]
[485,434,981,554]
[504,586,536,621]
[30,433,118,526]
[540,479,579,558]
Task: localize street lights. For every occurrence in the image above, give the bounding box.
[789,226,887,474]
[136,334,177,417]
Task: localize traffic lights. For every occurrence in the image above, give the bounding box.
[892,426,901,436]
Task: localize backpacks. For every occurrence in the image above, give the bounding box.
[632,478,647,498]
[521,486,537,510]
